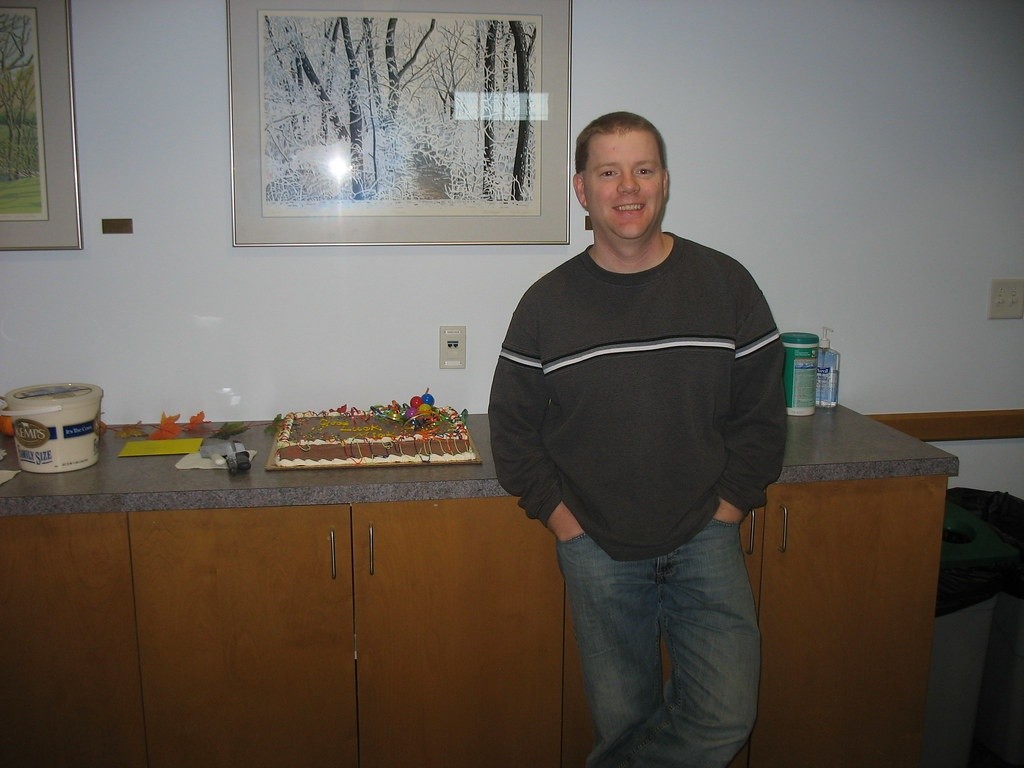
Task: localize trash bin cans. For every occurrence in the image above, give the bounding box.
[922,487,1024,768]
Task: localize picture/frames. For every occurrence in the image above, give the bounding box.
[0,0,84,250]
[228,0,571,245]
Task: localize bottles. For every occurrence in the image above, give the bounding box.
[780,332,819,415]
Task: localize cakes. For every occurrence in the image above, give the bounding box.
[274,387,477,467]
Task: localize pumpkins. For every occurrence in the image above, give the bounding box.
[0,396,15,437]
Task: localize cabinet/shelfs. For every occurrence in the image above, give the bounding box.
[0,473,948,768]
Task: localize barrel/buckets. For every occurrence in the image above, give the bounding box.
[0,383,104,473]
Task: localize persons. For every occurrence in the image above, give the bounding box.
[488,112,788,768]
